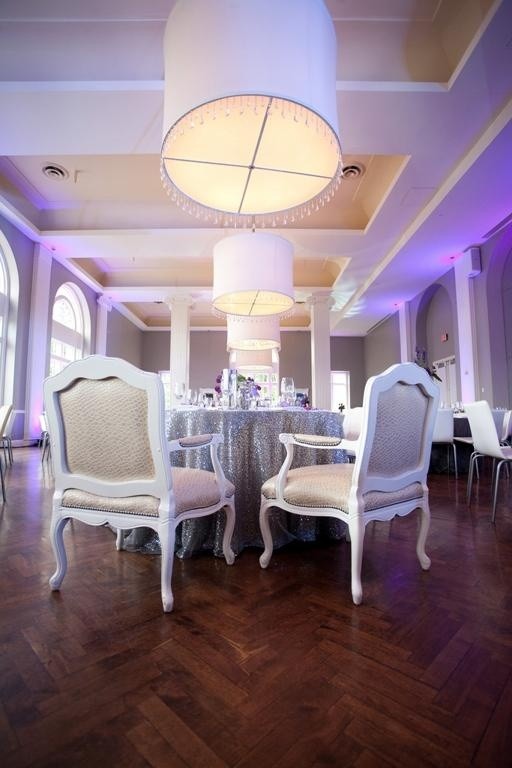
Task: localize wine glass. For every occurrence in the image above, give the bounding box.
[172,369,296,411]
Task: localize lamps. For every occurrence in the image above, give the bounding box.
[162,1,343,236]
[226,313,281,354]
[211,235,297,326]
[228,349,273,371]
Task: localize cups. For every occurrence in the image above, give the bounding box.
[440,399,463,414]
